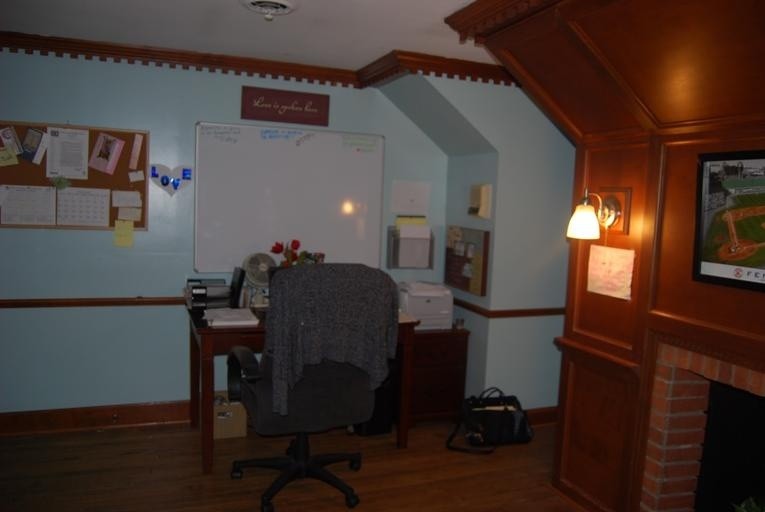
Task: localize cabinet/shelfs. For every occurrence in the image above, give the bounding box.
[412,325,469,422]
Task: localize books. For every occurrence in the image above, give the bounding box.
[88,133,125,174]
[394,213,431,268]
[204,308,261,327]
[399,281,450,299]
[17,127,51,164]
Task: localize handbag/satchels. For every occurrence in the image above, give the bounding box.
[458,383,535,450]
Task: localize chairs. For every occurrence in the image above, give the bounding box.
[226,258,403,512]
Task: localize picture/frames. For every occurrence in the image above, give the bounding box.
[694,148,764,292]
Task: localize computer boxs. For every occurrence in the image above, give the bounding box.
[353,370,394,436]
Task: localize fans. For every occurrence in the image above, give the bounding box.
[241,251,277,304]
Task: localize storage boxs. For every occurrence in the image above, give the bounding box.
[212,389,248,439]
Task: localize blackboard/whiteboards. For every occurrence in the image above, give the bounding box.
[194,121,385,272]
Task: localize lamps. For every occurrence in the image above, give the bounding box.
[567,186,621,242]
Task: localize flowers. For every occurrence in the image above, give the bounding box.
[270,239,309,268]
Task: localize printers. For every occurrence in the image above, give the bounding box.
[399,281,454,331]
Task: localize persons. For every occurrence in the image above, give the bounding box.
[2,128,13,139]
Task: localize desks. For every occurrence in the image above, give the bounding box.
[186,304,424,476]
